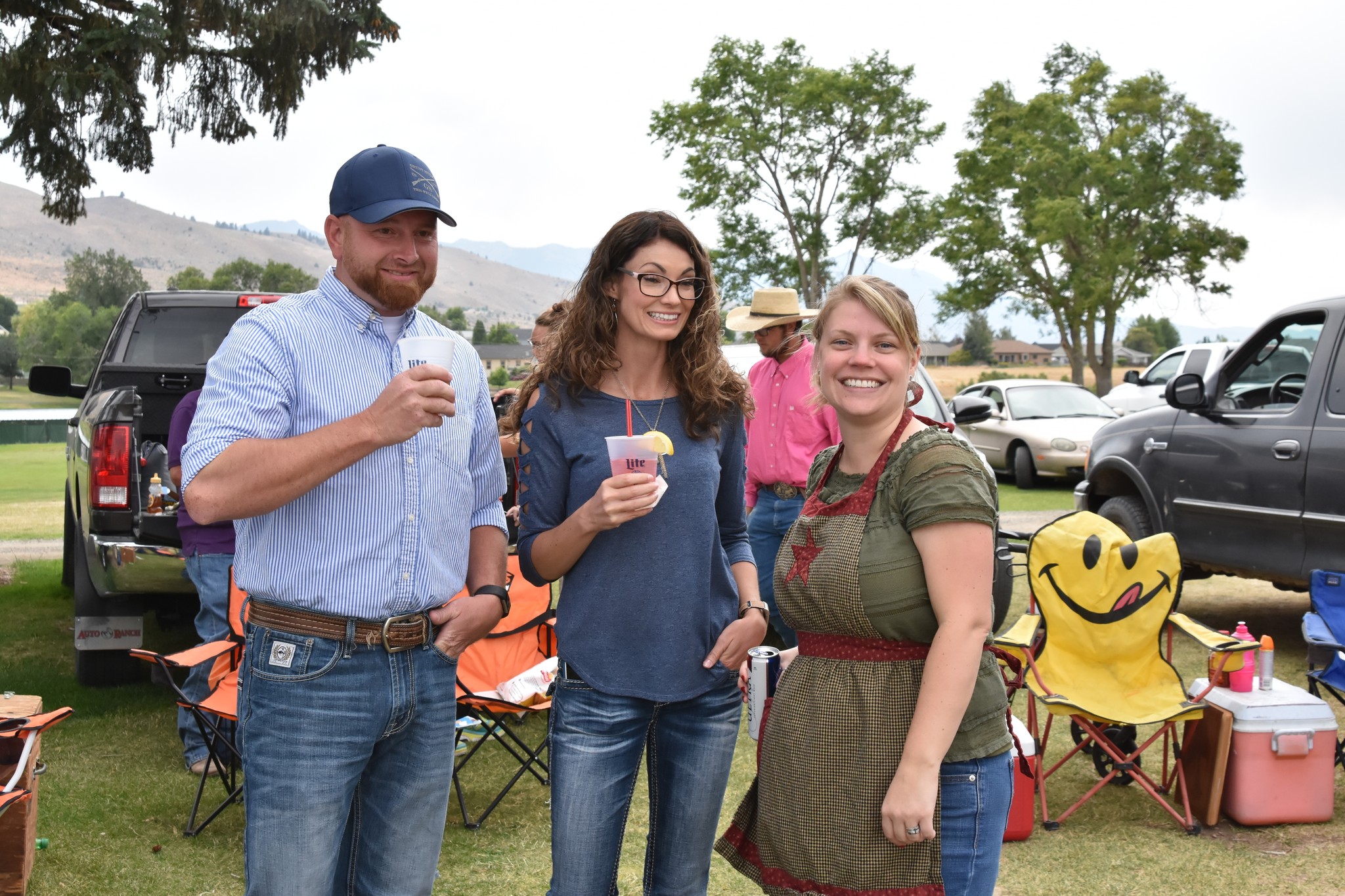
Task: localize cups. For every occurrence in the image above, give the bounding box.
[605,434,659,511]
[398,337,457,374]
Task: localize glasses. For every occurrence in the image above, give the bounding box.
[749,325,779,336]
[616,266,706,300]
[527,339,557,351]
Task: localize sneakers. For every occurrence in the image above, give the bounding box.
[455,713,506,742]
[455,741,467,756]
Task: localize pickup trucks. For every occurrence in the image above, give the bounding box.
[26,288,298,689]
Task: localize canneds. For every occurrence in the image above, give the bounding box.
[746,646,781,741]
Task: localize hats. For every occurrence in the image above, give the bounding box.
[725,287,823,331]
[329,144,457,227]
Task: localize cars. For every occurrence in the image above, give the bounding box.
[945,378,1123,490]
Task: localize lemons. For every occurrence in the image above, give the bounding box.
[638,431,674,455]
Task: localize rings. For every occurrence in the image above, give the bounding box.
[907,826,919,834]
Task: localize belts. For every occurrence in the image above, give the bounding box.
[767,483,801,499]
[248,602,445,654]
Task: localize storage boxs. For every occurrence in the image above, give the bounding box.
[1004,713,1038,844]
[0,693,43,896]
[1189,677,1339,827]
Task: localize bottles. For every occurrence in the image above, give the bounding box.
[1259,635,1275,691]
[147,473,163,514]
[1208,631,1229,688]
[1229,621,1255,692]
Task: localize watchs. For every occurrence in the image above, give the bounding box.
[738,599,770,625]
[472,584,510,619]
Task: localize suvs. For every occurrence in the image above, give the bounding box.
[1073,296,1345,612]
[1099,341,1314,417]
[720,341,997,490]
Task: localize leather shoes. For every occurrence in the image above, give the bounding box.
[191,756,227,777]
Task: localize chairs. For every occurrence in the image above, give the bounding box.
[1300,568,1345,769]
[127,564,252,838]
[994,509,1261,836]
[0,706,77,817]
[442,552,558,831]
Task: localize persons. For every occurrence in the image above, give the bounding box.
[490,213,768,896]
[726,287,844,651]
[167,387,237,776]
[711,274,1013,896]
[179,143,511,896]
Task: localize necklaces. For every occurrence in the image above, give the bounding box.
[614,363,673,479]
